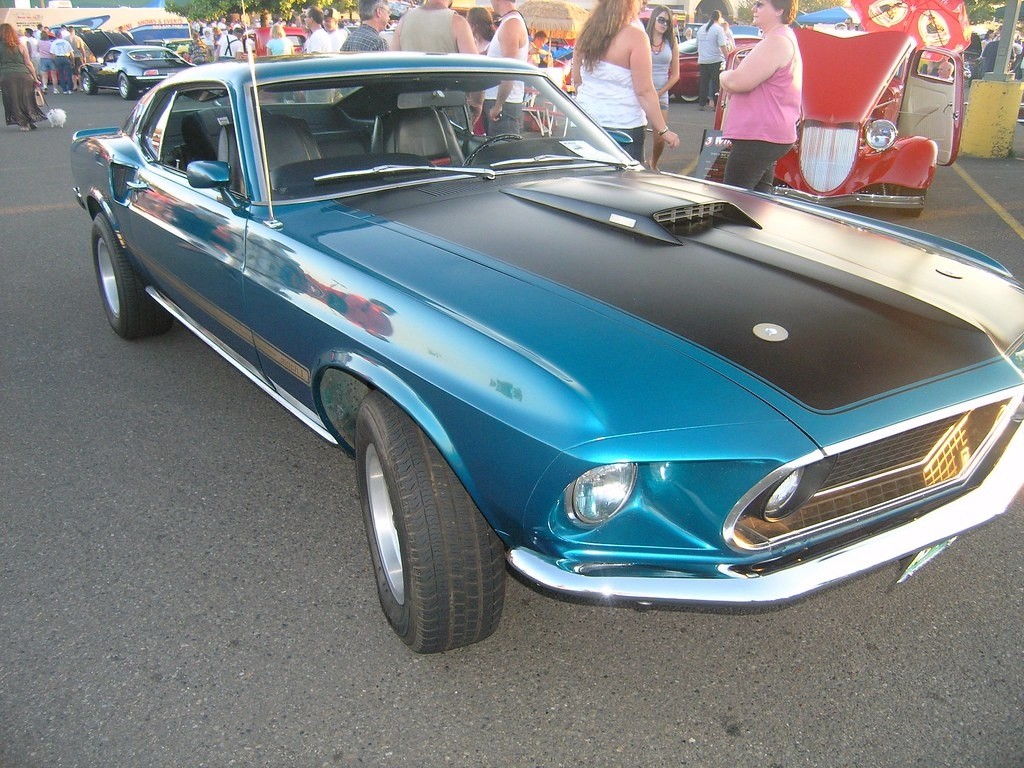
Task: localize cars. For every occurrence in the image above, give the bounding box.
[69,52,1024,654]
[695,31,966,220]
[246,26,309,55]
[77,31,197,100]
[669,31,766,102]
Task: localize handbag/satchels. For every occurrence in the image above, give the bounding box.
[35,90,44,107]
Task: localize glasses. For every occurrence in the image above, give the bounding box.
[376,6,392,17]
[753,2,762,8]
[656,16,670,26]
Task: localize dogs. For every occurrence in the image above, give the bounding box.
[47,109,68,128]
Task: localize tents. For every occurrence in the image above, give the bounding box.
[796,6,861,26]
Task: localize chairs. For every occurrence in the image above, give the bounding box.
[365,92,465,168]
[218,111,323,194]
[522,87,568,138]
[182,106,271,162]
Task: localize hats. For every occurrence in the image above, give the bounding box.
[323,8,338,19]
[233,24,244,34]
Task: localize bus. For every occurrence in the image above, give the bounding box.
[0,6,195,66]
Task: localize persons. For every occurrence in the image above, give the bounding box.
[300,4,349,53]
[391,0,485,134]
[467,0,530,138]
[259,8,303,27]
[682,9,741,112]
[23,23,87,95]
[834,18,864,32]
[717,0,803,194]
[571,0,680,165]
[523,30,552,102]
[266,23,295,56]
[0,23,50,131]
[190,17,256,62]
[642,5,679,170]
[919,25,1024,84]
[340,0,390,51]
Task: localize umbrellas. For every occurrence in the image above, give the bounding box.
[851,0,972,52]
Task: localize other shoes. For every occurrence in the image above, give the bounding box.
[73,88,77,91]
[68,90,73,94]
[43,85,47,95]
[54,89,60,95]
[63,92,68,94]
[79,89,83,93]
[699,105,706,110]
[30,123,37,129]
[21,125,29,132]
